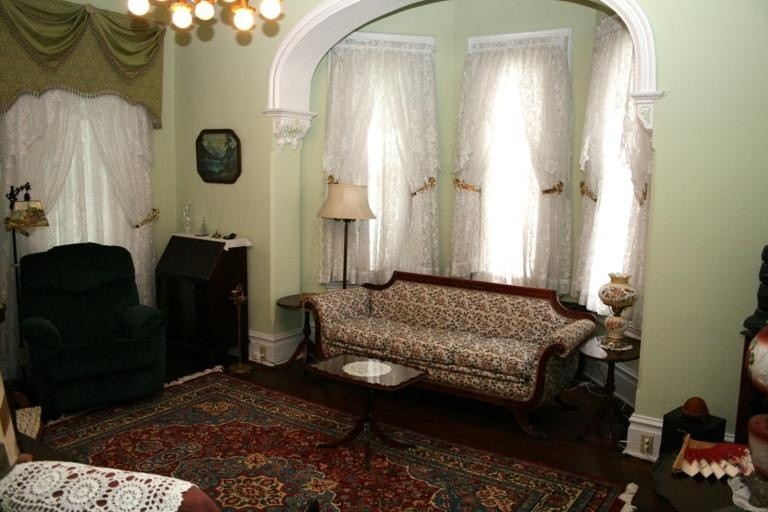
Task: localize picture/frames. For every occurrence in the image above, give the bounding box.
[196,128,240,184]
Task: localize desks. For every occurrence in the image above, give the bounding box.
[275,291,333,368]
[650,451,752,512]
[575,334,643,451]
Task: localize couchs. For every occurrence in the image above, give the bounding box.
[301,272,598,406]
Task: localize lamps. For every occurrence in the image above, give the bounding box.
[127,1,281,36]
[316,180,377,289]
[596,270,640,352]
[4,182,49,404]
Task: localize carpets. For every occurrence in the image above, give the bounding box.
[18,366,638,511]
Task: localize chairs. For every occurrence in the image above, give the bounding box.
[17,242,161,413]
[0,460,218,511]
